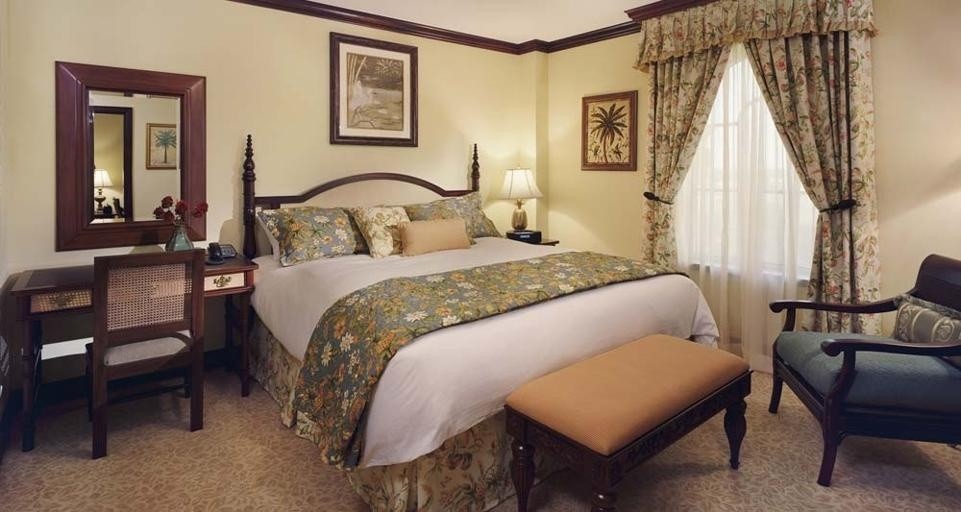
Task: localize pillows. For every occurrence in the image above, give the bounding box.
[254,212,288,262]
[405,192,502,238]
[349,206,411,258]
[894,293,961,367]
[399,218,472,256]
[255,207,369,267]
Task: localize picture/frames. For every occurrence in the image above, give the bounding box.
[330,32,418,148]
[146,123,179,169]
[580,91,639,171]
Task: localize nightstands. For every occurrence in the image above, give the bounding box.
[539,238,560,246]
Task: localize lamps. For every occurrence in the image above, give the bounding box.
[498,169,542,231]
[93,169,114,213]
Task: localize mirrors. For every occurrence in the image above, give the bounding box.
[56,60,208,250]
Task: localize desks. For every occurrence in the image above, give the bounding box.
[94,209,118,219]
[9,254,259,451]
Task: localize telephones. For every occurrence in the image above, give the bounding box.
[207,242,237,259]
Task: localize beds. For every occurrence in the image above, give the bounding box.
[241,133,718,510]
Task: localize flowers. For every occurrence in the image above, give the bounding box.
[153,197,208,225]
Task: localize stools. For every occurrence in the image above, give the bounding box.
[504,335,755,511]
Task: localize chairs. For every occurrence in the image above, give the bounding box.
[112,198,125,218]
[769,254,961,486]
[85,249,205,459]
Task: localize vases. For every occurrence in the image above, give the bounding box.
[165,222,194,252]
